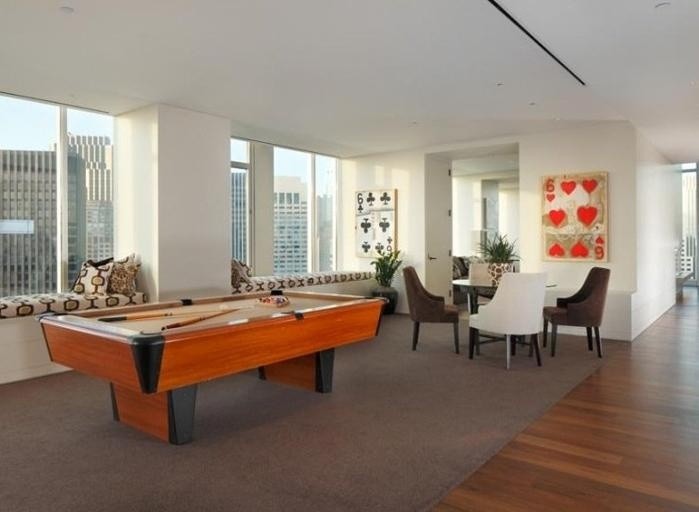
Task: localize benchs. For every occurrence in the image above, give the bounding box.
[0,293,144,386]
[235,268,376,298]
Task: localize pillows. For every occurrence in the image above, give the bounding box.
[71,261,113,296]
[114,253,137,288]
[70,257,113,291]
[108,260,141,297]
[231,256,255,294]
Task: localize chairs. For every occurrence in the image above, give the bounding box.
[542,266,610,358]
[468,272,545,367]
[402,266,460,355]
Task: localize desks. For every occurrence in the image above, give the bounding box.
[32,288,391,445]
[450,279,547,359]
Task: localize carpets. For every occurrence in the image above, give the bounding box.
[0,311,629,512]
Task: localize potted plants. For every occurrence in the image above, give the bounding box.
[475,233,521,281]
[369,249,402,315]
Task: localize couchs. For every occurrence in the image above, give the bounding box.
[452,255,485,305]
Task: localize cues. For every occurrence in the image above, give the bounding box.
[95,309,239,330]
